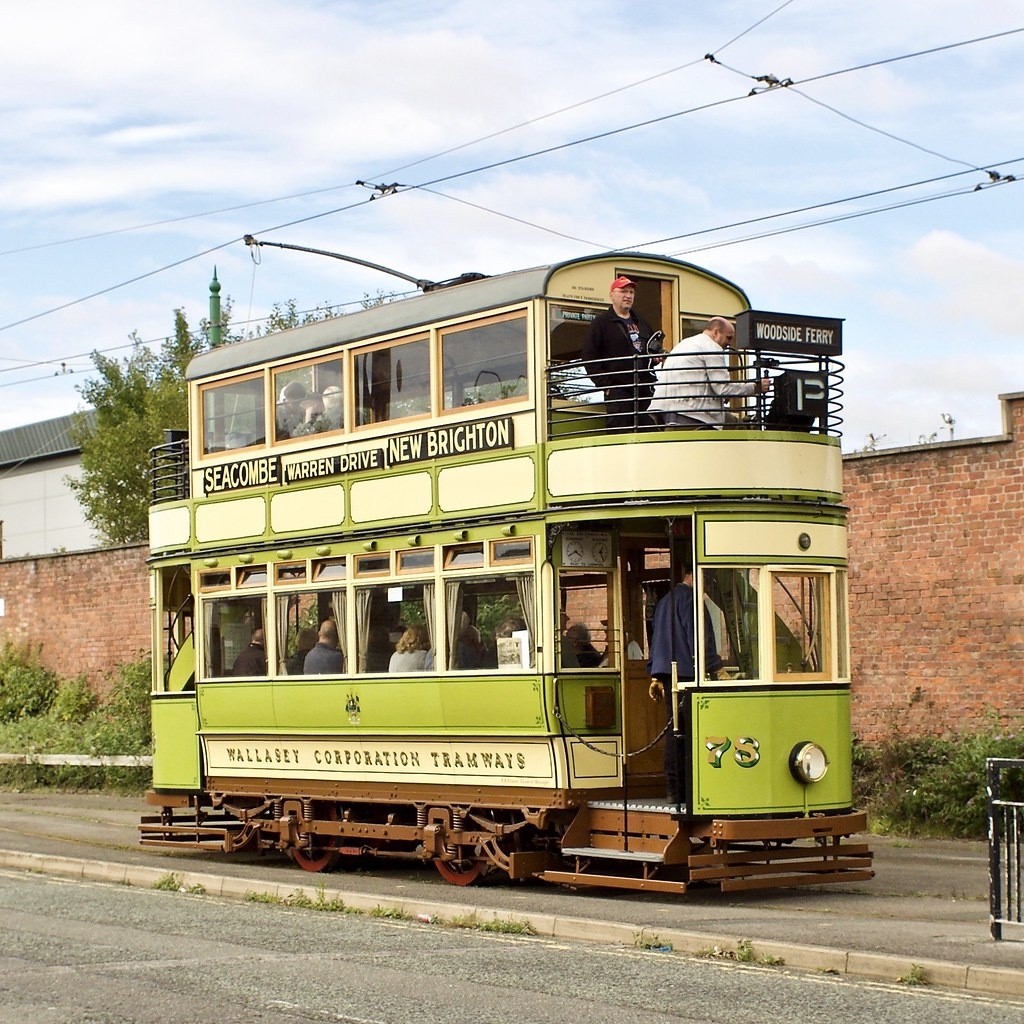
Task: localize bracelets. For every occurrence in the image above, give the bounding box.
[650,677,661,682]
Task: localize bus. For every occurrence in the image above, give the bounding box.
[138,233,875,893]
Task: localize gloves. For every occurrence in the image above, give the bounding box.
[716,669,731,680]
[649,677,665,701]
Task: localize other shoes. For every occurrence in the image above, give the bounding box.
[666,792,684,804]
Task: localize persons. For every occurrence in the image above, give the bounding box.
[645,314,772,431]
[203,380,366,455]
[645,562,745,804]
[230,606,643,678]
[582,275,670,434]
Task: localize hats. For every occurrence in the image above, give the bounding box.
[682,560,717,584]
[600,619,625,627]
[610,275,637,292]
[559,608,567,614]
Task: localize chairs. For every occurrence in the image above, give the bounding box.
[461,627,491,666]
[368,627,404,673]
[287,628,319,675]
